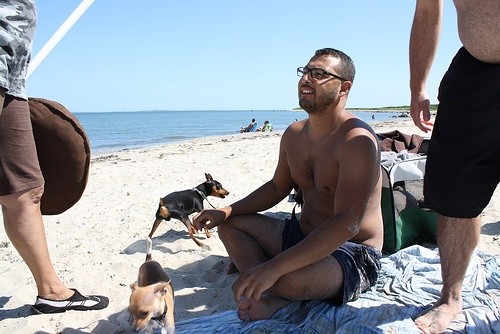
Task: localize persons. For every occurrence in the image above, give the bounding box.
[241,118,256,133]
[0,0,109,316]
[407,1,500,333]
[193,47,384,323]
[257,120,269,131]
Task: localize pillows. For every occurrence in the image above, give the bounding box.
[28,97,91,215]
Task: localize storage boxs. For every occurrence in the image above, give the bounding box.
[376,130,438,255]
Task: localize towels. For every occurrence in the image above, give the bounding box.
[375,129,424,154]
[380,149,410,167]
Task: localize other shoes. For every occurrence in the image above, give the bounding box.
[34,289,110,314]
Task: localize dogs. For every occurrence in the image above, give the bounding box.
[127,235,176,334]
[148,173,230,251]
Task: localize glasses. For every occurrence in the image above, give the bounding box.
[297,67,348,82]
